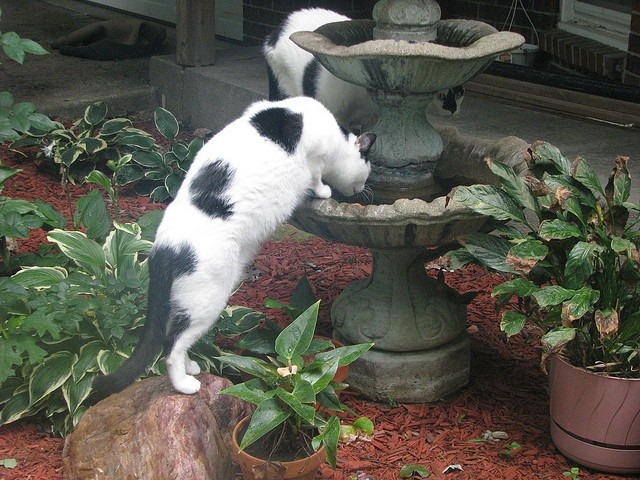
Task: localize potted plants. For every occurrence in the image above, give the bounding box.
[254,311,353,431]
[452,142,640,472]
[212,311,373,475]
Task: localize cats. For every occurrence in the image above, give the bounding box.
[262,7,465,119]
[92,97,377,398]
